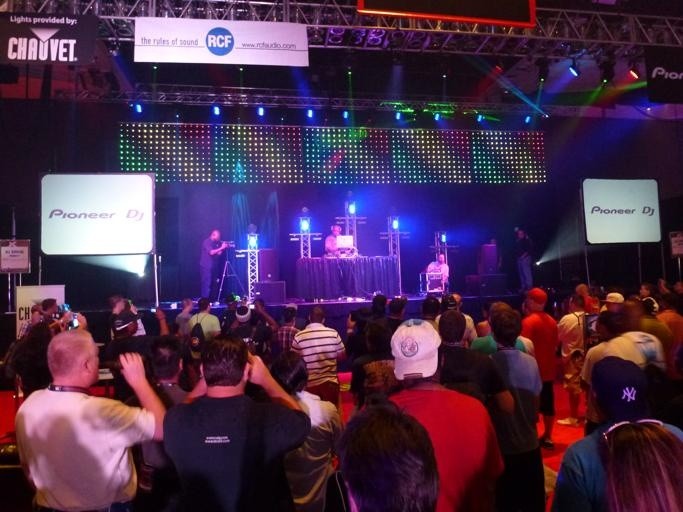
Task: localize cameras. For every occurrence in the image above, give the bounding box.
[216,240,236,249]
[104,356,160,390]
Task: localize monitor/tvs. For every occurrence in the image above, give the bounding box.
[583,179,661,244]
[337,236,353,248]
[40,174,154,254]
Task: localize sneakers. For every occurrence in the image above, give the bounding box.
[558,416,579,426]
[584,416,588,425]
[539,437,553,448]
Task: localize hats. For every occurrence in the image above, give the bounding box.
[590,356,644,420]
[602,293,626,304]
[391,320,441,381]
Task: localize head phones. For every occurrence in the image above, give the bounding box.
[642,297,658,316]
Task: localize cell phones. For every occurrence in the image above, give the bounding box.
[151,308,156,312]
[127,300,132,306]
[72,313,79,328]
[249,306,254,308]
[350,311,363,321]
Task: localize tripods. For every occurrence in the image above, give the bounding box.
[217,248,247,304]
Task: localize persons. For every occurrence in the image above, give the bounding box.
[272,307,300,350]
[249,302,280,354]
[439,347,546,511]
[325,225,343,253]
[658,279,682,315]
[336,409,440,512]
[4,297,89,393]
[574,277,661,314]
[515,229,532,288]
[270,350,343,511]
[337,310,370,407]
[200,230,229,298]
[435,311,514,428]
[290,306,345,408]
[428,252,449,295]
[366,294,388,330]
[360,318,504,512]
[520,288,562,451]
[640,296,670,355]
[15,330,166,511]
[109,296,141,338]
[229,306,260,341]
[556,294,591,425]
[140,335,191,489]
[657,293,682,416]
[471,303,534,356]
[598,419,682,512]
[163,334,311,511]
[488,308,541,412]
[421,297,442,333]
[617,302,665,370]
[163,367,201,404]
[222,295,240,333]
[187,298,220,378]
[368,298,408,360]
[580,311,646,435]
[549,356,681,511]
[435,295,479,348]
